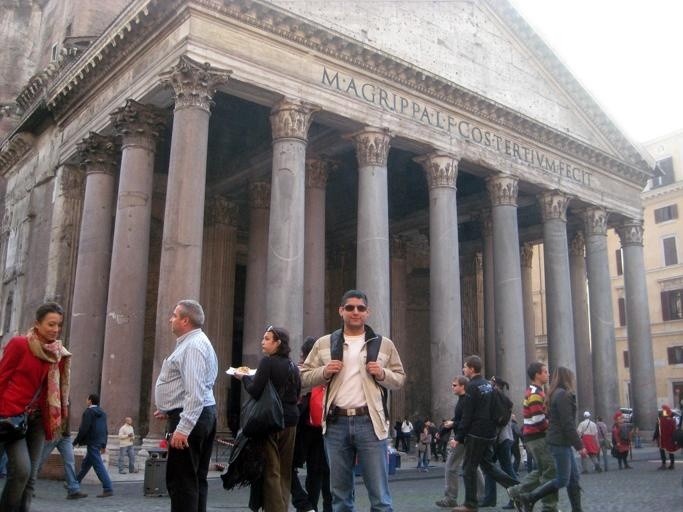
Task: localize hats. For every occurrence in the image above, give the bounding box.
[491,376,502,384]
[584,412,591,418]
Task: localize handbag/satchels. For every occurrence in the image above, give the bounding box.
[603,439,611,450]
[241,378,285,438]
[0,412,29,443]
[227,436,265,483]
[612,447,617,457]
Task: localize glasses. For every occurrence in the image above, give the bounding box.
[344,305,367,312]
[453,385,459,387]
[265,325,280,339]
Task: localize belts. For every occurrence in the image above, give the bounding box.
[334,406,368,416]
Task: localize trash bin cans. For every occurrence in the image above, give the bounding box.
[389,449,401,475]
[142,448,169,497]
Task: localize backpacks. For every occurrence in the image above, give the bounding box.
[309,385,324,427]
[490,388,513,427]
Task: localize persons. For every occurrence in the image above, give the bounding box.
[151,298,219,511]
[653,403,681,470]
[118,417,139,474]
[393,353,632,511]
[299,287,407,511]
[0,301,72,512]
[219,324,333,511]
[32,399,88,500]
[63,394,113,499]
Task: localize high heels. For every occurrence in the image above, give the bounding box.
[624,464,631,469]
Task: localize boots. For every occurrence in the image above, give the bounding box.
[520,480,558,512]
[567,480,582,512]
[669,464,674,470]
[658,464,665,469]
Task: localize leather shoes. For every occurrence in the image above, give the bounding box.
[478,499,496,507]
[502,499,514,509]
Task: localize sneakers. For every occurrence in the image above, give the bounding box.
[130,470,138,473]
[66,491,88,499]
[96,491,113,498]
[436,497,458,507]
[507,485,522,512]
[451,506,478,512]
[119,471,126,474]
